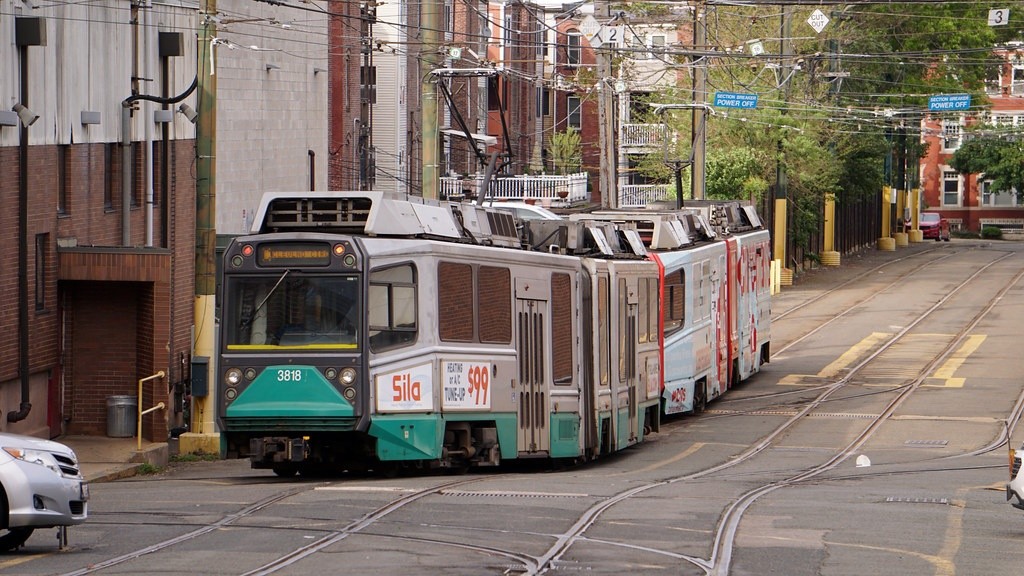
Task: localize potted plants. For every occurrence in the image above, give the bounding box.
[525,198,535,206]
[499,198,508,202]
[557,187,570,199]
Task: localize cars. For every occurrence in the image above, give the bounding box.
[0,429,90,555]
[902,212,951,242]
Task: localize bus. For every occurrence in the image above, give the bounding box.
[215,191,665,480]
[578,198,772,418]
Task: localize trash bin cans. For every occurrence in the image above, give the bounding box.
[104,394,137,438]
